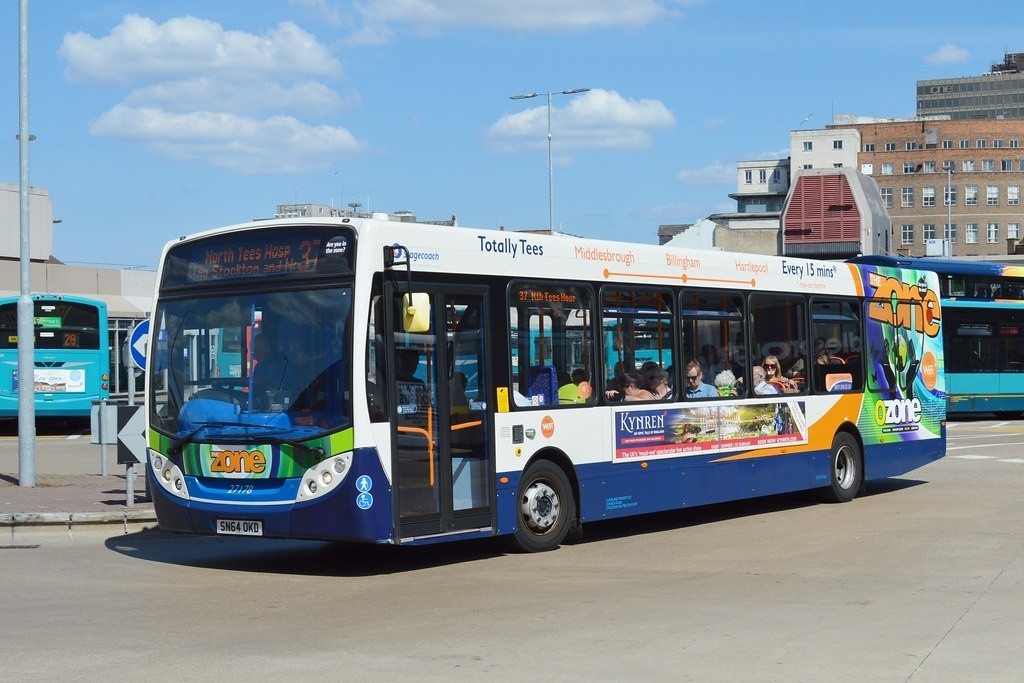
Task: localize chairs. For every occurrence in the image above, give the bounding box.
[766,380,787,394]
[844,355,861,365]
[791,377,805,393]
[829,356,846,366]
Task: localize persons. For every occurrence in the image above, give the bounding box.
[395,348,428,404]
[449,373,467,406]
[753,337,830,394]
[34,318,44,328]
[556,369,594,404]
[602,359,674,402]
[253,333,299,400]
[683,343,746,398]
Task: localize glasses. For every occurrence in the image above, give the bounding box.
[686,374,699,381]
[764,364,776,369]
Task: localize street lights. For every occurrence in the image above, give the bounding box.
[508,87,593,235]
[942,165,956,261]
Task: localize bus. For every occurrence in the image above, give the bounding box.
[0,292,110,427]
[212,308,675,402]
[840,252,1024,421]
[141,213,949,556]
